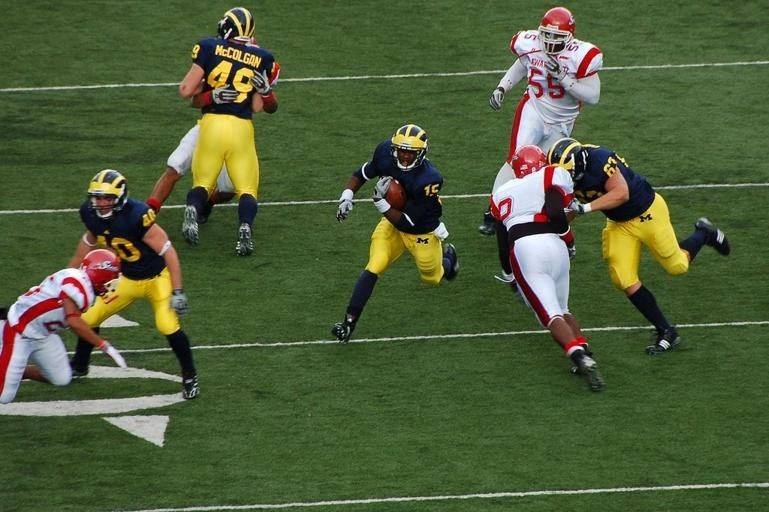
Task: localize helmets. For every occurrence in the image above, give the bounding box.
[513,138,582,178]
[391,124,427,171]
[539,7,575,37]
[81,249,121,295]
[217,7,255,41]
[87,169,128,210]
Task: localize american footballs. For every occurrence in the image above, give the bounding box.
[386,180,406,212]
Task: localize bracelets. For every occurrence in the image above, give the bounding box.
[584,203,592,214]
[373,199,391,214]
[339,189,354,202]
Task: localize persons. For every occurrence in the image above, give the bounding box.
[329,124,459,341]
[180,8,280,254]
[0,249,127,404]
[547,139,730,354]
[145,38,278,224]
[491,144,606,393]
[479,8,604,236]
[68,168,200,399]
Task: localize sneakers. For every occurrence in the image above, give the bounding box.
[646,326,679,352]
[695,217,729,255]
[183,373,199,399]
[443,243,459,281]
[480,220,494,235]
[235,223,253,256]
[182,205,198,243]
[570,344,605,391]
[331,322,352,342]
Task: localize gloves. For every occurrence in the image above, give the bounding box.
[372,176,393,199]
[545,51,565,82]
[489,87,504,110]
[212,84,237,104]
[250,70,270,96]
[171,289,187,314]
[568,197,584,214]
[336,199,353,223]
[569,245,576,259]
